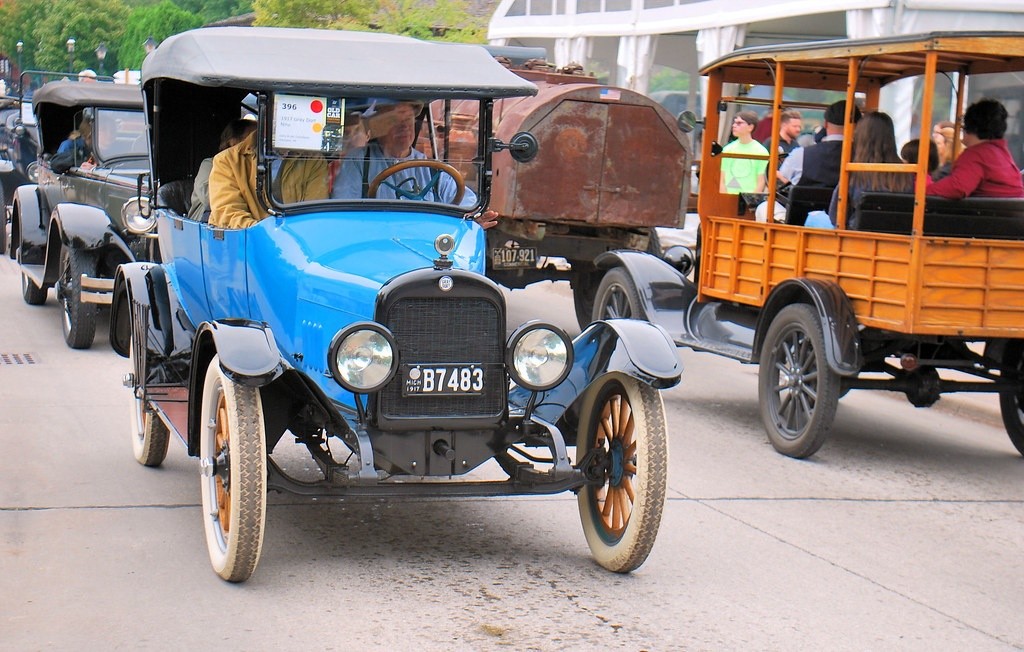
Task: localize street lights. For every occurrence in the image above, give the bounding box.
[66,37,76,73]
[16,40,24,81]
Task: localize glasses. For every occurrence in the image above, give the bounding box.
[732,120,745,127]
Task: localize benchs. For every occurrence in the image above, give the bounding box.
[854,191,1024,241]
[157,180,194,219]
[783,185,836,226]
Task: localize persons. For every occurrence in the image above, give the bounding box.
[828,112,915,229]
[765,111,803,191]
[751,106,785,143]
[331,95,499,230]
[718,110,770,195]
[901,139,940,189]
[925,97,1024,198]
[208,97,329,230]
[327,114,371,196]
[755,98,870,224]
[187,119,258,223]
[0,70,97,100]
[50,113,116,175]
[932,122,963,183]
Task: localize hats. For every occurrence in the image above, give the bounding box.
[823,100,862,125]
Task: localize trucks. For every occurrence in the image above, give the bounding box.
[415,45,694,330]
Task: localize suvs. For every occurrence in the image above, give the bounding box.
[123,27,684,582]
[590,30,1024,459]
[0,70,158,350]
